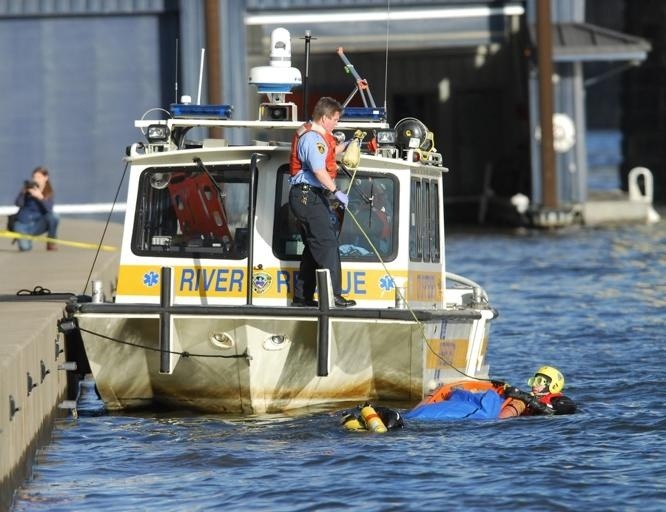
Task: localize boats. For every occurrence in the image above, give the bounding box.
[66,27,498,417]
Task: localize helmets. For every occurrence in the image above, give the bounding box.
[535,366,565,395]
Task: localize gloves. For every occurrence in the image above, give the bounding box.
[335,191,349,209]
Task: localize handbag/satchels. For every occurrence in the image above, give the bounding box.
[8,215,19,231]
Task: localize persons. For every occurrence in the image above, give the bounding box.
[8,166,60,252]
[527,365,575,413]
[287,96,358,308]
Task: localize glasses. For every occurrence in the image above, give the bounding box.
[528,377,549,387]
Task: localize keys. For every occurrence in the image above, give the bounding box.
[300,189,309,205]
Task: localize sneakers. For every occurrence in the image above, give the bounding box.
[48,242,57,250]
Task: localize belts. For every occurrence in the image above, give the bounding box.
[310,187,330,195]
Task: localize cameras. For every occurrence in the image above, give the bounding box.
[24,180,39,189]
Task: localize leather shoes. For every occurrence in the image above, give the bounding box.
[334,296,356,307]
[291,297,318,307]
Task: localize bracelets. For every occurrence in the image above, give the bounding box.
[333,187,340,196]
